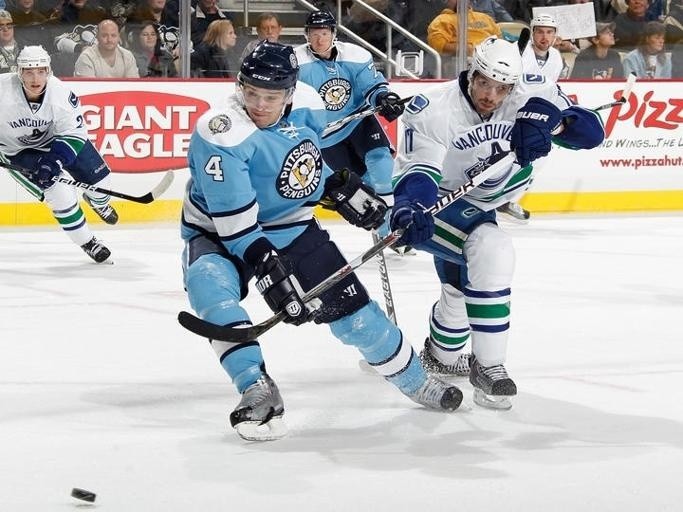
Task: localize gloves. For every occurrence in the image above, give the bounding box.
[26,152,64,191]
[321,168,389,232]
[375,91,405,122]
[391,200,435,245]
[510,96,562,167]
[247,244,322,326]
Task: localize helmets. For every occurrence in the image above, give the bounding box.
[16,45,51,71]
[303,9,337,36]
[467,36,522,92]
[532,14,560,37]
[234,39,300,111]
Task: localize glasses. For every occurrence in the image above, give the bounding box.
[1,23,13,29]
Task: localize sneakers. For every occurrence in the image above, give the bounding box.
[80,236,111,264]
[418,337,468,377]
[498,203,530,220]
[230,372,284,428]
[389,245,416,255]
[82,193,119,225]
[409,375,464,414]
[469,353,516,396]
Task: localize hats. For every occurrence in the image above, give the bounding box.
[587,19,617,39]
[0,9,12,19]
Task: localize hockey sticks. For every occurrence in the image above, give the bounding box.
[0,163,174,204]
[178,147,518,342]
[551,71,638,135]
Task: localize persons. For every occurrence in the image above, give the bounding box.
[391,34,603,395]
[180,40,464,427]
[1,1,238,80]
[292,11,413,254]
[296,1,682,80]
[0,45,118,262]
